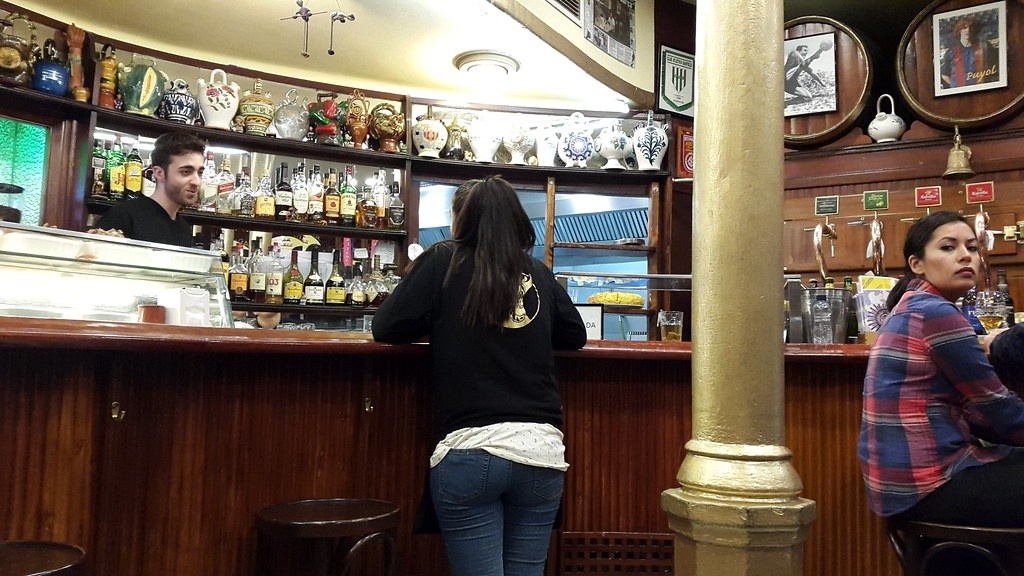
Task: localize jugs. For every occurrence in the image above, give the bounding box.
[867,93,906,143]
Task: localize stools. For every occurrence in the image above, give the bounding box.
[255,498,402,576]
[0,540,87,576]
[884,518,1024,576]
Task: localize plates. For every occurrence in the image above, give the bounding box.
[78,312,131,322]
[606,304,643,310]
[0,305,63,318]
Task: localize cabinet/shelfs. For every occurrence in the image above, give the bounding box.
[0,0,670,341]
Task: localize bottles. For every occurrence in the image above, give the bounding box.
[962,285,984,336]
[90,50,406,309]
[410,101,670,171]
[807,275,854,289]
[994,269,1015,328]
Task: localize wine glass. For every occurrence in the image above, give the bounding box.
[975,290,1006,335]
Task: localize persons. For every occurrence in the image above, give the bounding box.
[96,131,206,247]
[372,174,587,576]
[861,210,1024,576]
[230,310,308,330]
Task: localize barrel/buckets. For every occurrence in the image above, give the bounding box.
[799,288,852,344]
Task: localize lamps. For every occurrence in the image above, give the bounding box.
[452,51,521,76]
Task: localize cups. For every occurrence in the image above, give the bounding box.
[812,295,833,345]
[657,310,684,342]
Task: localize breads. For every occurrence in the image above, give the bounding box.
[588,292,644,306]
